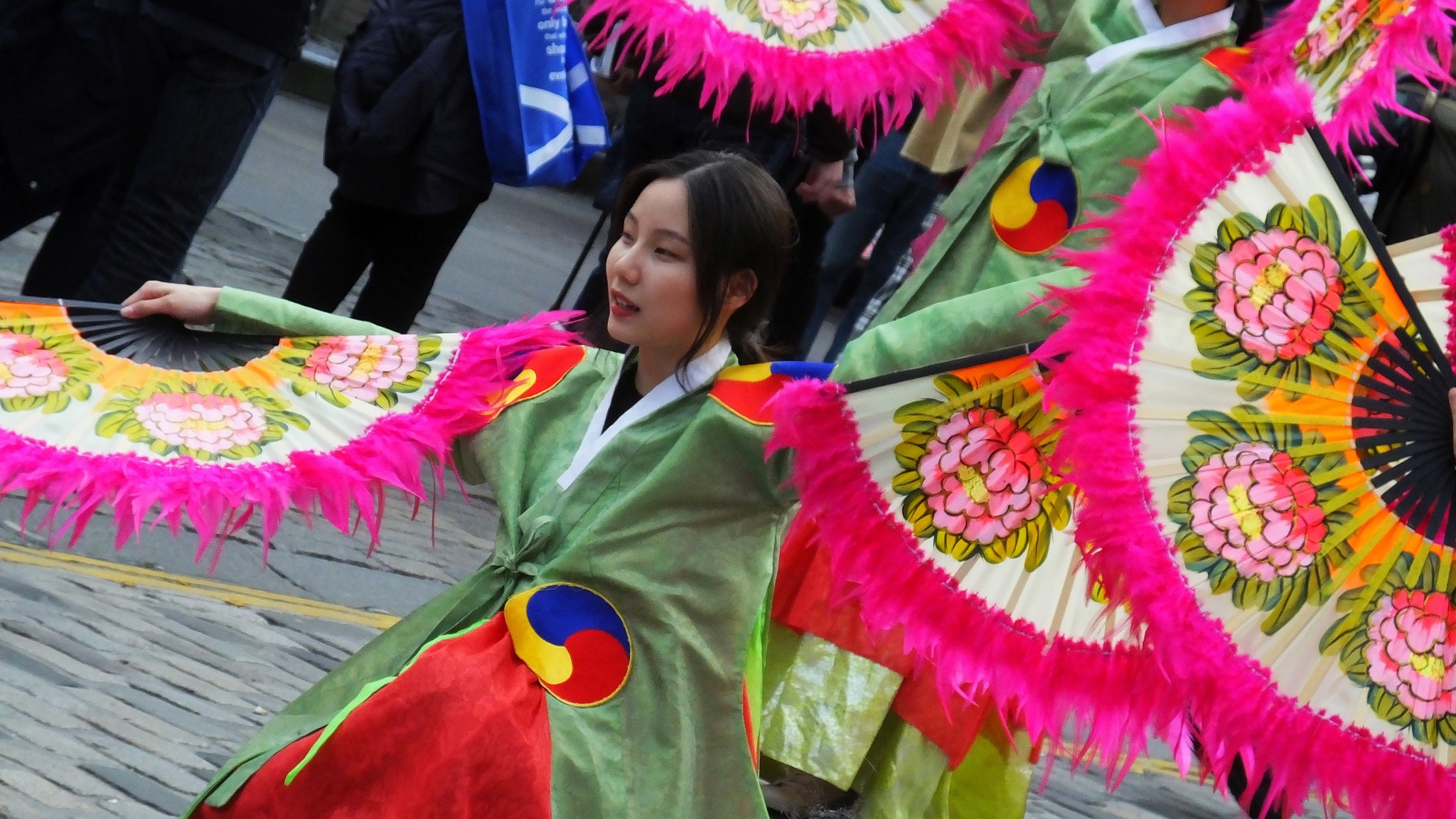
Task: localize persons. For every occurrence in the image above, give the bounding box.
[757,1,1271,819]
[0,0,1451,365]
[115,147,1095,818]
